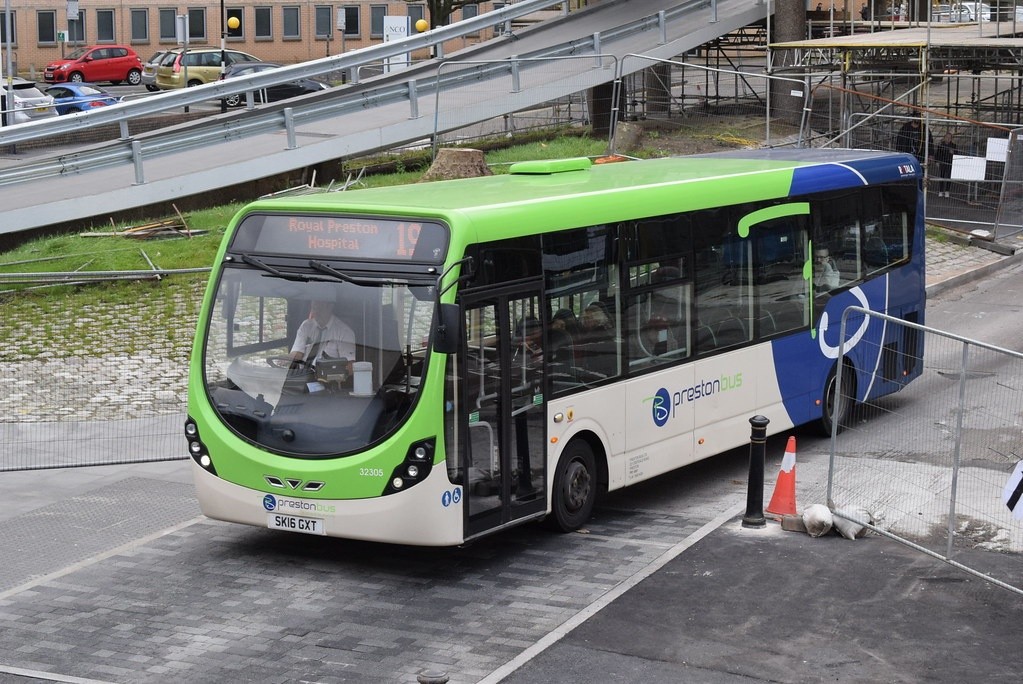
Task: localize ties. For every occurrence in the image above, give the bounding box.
[303,327,327,370]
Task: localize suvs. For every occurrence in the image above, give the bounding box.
[1,76,60,128]
[155,47,265,91]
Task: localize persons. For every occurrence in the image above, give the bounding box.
[935,132,958,197]
[828,4,836,11]
[791,246,840,304]
[816,3,822,11]
[519,316,543,358]
[580,305,617,378]
[859,3,867,21]
[288,300,356,376]
[552,316,582,364]
[629,321,680,358]
[896,113,935,162]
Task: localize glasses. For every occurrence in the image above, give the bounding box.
[813,255,828,259]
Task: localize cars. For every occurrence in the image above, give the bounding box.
[214,61,333,108]
[42,43,144,86]
[42,81,124,116]
[952,2,990,22]
[140,47,232,93]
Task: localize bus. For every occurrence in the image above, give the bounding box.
[183,140,930,551]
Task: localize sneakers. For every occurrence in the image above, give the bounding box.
[938,191,944,198]
[945,191,950,198]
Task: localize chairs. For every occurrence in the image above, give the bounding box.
[515,235,890,382]
[335,313,368,362]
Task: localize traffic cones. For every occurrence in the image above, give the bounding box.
[762,434,799,519]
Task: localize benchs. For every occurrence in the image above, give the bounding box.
[823,30,842,38]
[757,31,767,46]
[733,19,764,43]
